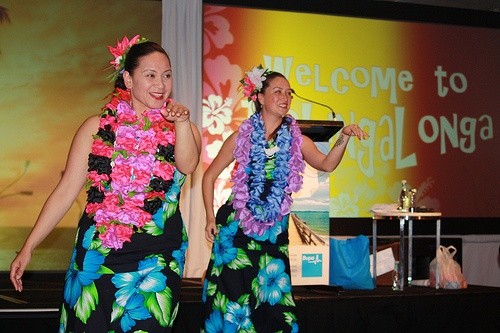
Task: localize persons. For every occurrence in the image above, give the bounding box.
[10,41,202,333]
[201,71,370,333]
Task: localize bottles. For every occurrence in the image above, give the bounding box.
[392,261,403,291]
[400,180,410,211]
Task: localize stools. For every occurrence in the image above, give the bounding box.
[462,233,500,287]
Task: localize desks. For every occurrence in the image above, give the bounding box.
[371,210,442,291]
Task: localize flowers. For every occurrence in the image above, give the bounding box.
[239,64,271,102]
[101,35,149,83]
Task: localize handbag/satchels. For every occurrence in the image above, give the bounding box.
[429,245,468,288]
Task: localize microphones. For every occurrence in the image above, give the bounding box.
[291,89,337,121]
